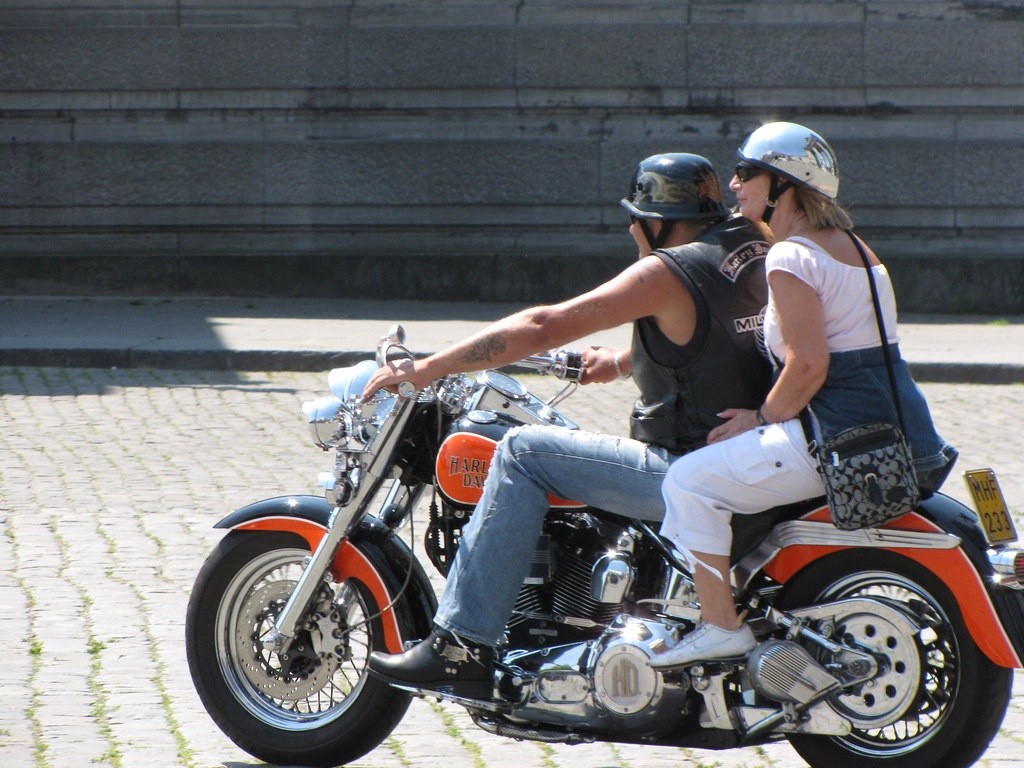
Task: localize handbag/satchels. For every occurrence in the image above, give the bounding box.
[813,422,920,532]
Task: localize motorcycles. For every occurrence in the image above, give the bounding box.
[184,324,1024,768]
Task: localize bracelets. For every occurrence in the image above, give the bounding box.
[615,356,631,382]
[757,411,769,427]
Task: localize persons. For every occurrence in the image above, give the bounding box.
[362,121,959,700]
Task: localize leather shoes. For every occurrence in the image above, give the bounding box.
[366,625,495,699]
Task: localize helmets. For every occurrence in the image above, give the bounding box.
[616,152,731,220]
[736,120,840,199]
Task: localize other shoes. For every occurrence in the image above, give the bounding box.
[646,621,757,671]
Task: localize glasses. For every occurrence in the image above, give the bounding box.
[734,163,767,183]
[629,212,637,224]
